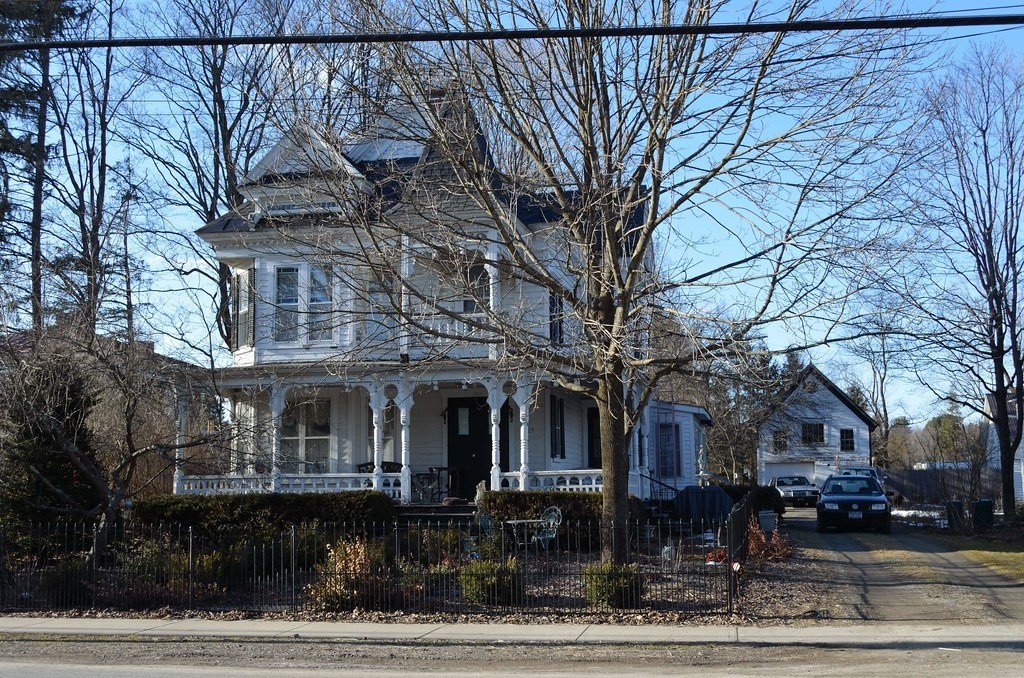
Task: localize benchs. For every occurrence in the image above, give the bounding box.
[358,463,436,502]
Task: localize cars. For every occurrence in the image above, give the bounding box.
[768,474,820,508]
[812,474,895,534]
[838,466,888,494]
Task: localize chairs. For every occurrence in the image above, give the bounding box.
[859,487,873,494]
[831,485,843,492]
[528,506,562,564]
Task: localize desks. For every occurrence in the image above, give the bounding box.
[429,467,461,502]
[507,520,546,565]
